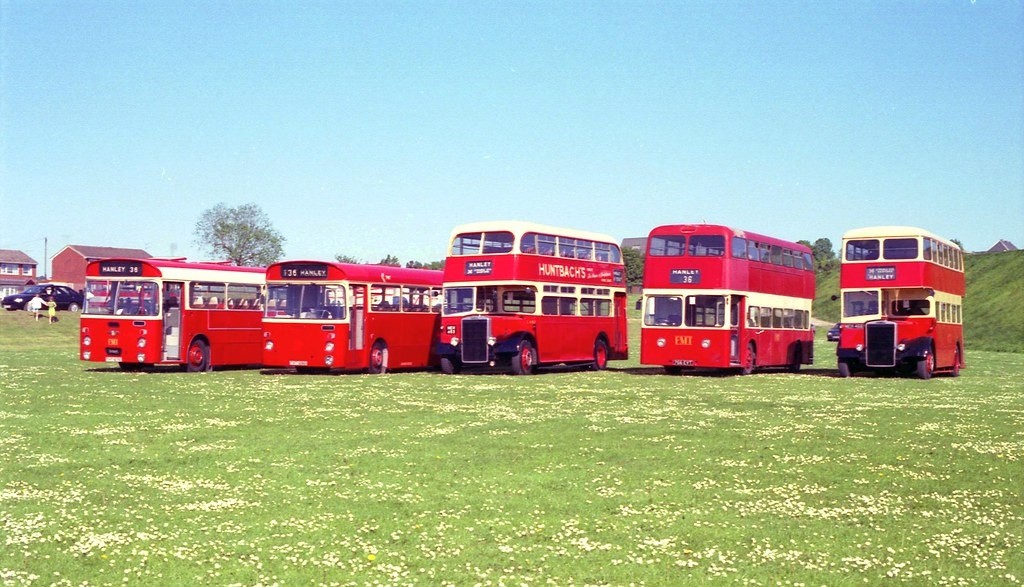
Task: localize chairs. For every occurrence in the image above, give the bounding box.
[865,301,886,314]
[485,247,605,262]
[755,316,801,327]
[191,296,287,310]
[852,301,863,315]
[378,300,439,312]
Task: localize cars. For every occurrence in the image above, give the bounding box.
[1,284,83,312]
[827,323,841,341]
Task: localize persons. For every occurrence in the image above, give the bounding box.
[46,297,57,325]
[28,294,47,321]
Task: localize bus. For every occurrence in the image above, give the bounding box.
[259,260,443,375]
[78,256,265,374]
[638,224,814,374]
[439,222,629,377]
[835,225,967,379]
